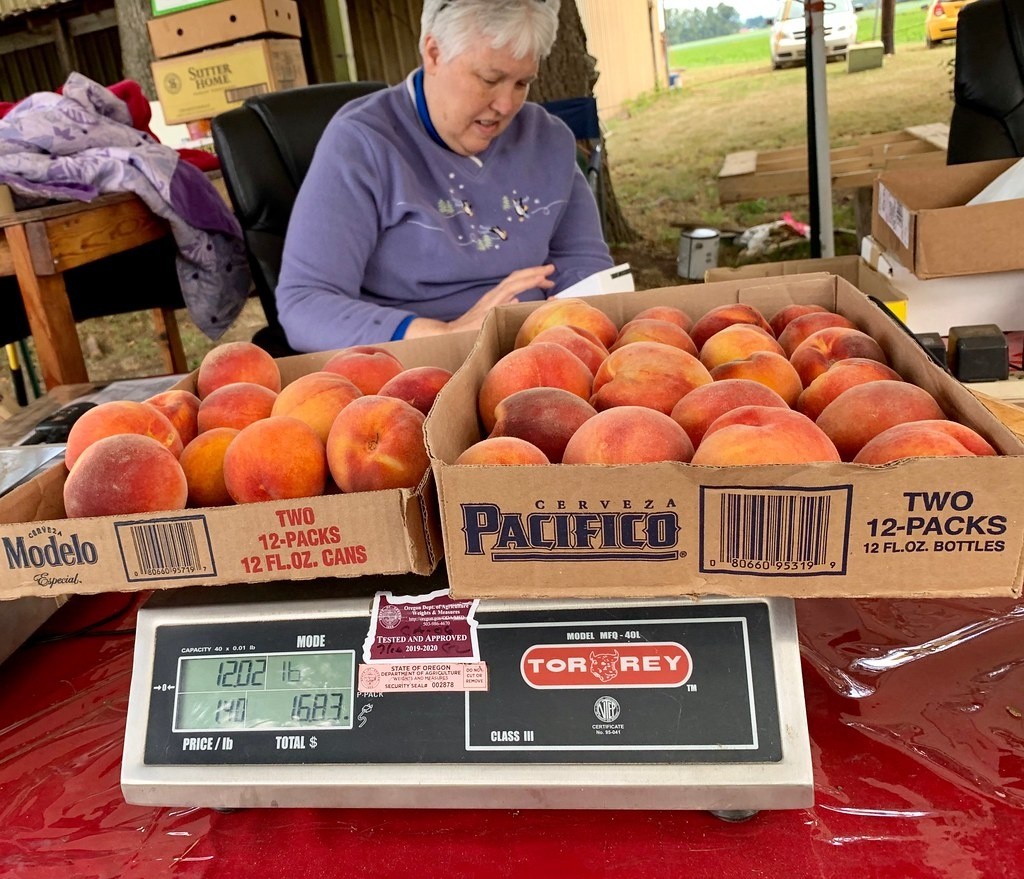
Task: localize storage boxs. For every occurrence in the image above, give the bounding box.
[0,331,479,601]
[706,253,908,327]
[422,274,1023,602]
[148,0,308,125]
[869,156,1024,282]
[861,235,1023,338]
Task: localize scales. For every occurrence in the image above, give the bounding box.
[121,557,813,822]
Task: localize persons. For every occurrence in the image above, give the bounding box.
[274,1,612,352]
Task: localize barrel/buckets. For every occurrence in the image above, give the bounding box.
[677,229,719,280]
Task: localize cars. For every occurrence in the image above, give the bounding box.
[767,0,863,70]
[919,0,981,49]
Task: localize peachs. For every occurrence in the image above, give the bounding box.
[63,339,455,518]
[452,297,998,466]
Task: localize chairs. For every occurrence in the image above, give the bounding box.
[208,81,389,353]
[948,0,1024,163]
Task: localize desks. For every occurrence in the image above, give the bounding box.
[1,332,1024,879]
[0,168,229,390]
